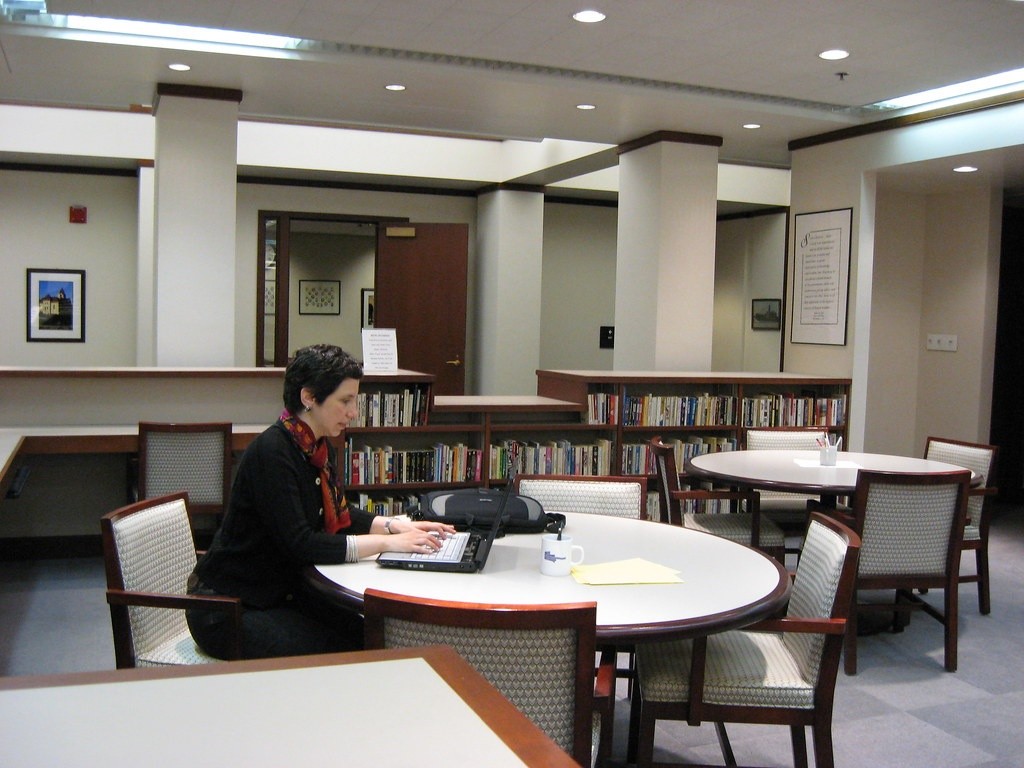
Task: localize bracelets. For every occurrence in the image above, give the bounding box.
[384,517,401,534]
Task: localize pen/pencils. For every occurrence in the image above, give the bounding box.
[836,436,842,447]
[825,431,830,447]
[816,439,822,447]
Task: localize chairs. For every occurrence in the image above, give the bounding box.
[803,470,972,677]
[514,472,648,524]
[904,436,998,624]
[99,492,242,670]
[623,513,862,768]
[742,426,837,519]
[650,436,785,569]
[119,420,233,537]
[361,589,617,768]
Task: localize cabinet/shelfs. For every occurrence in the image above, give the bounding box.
[345,370,435,428]
[535,368,852,527]
[341,421,618,522]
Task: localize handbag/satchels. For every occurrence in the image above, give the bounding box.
[405,487,566,540]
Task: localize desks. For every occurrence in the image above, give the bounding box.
[434,392,584,424]
[302,513,793,766]
[685,448,984,518]
[1,645,582,768]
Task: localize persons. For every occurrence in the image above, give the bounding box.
[185,343,457,661]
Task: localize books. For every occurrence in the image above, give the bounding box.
[645,492,731,523]
[349,492,426,518]
[589,391,847,427]
[345,386,429,427]
[342,435,747,485]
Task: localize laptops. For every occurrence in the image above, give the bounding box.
[375,467,517,573]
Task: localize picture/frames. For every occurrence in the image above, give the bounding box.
[26,269,86,343]
[298,278,342,317]
[361,288,375,331]
[752,298,783,331]
